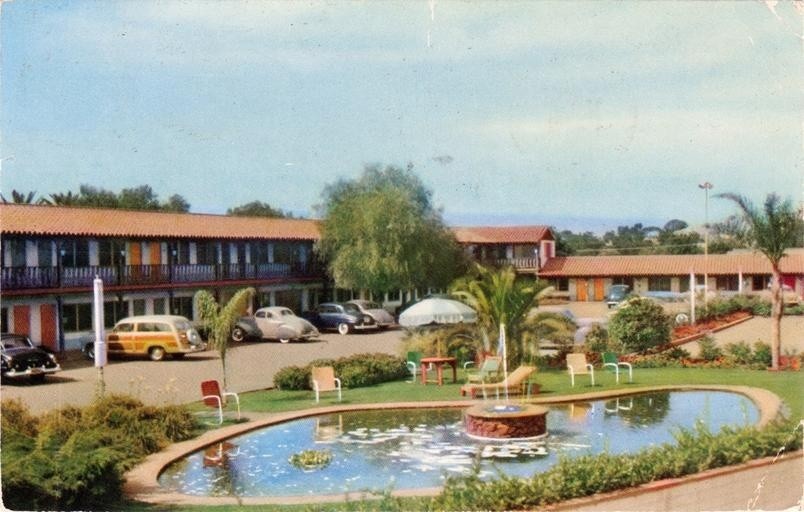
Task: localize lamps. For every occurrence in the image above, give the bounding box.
[314,414,344,442]
[460,364,538,399]
[565,353,595,387]
[464,352,492,374]
[408,351,431,384]
[204,442,239,467]
[570,403,593,422]
[605,398,633,419]
[200,380,240,425]
[601,352,633,384]
[469,357,501,383]
[312,366,341,403]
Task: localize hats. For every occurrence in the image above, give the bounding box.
[303,303,375,336]
[1,335,61,381]
[228,305,319,344]
[348,300,395,328]
[606,284,630,309]
[84,315,208,361]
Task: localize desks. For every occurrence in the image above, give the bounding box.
[697,181,714,313]
